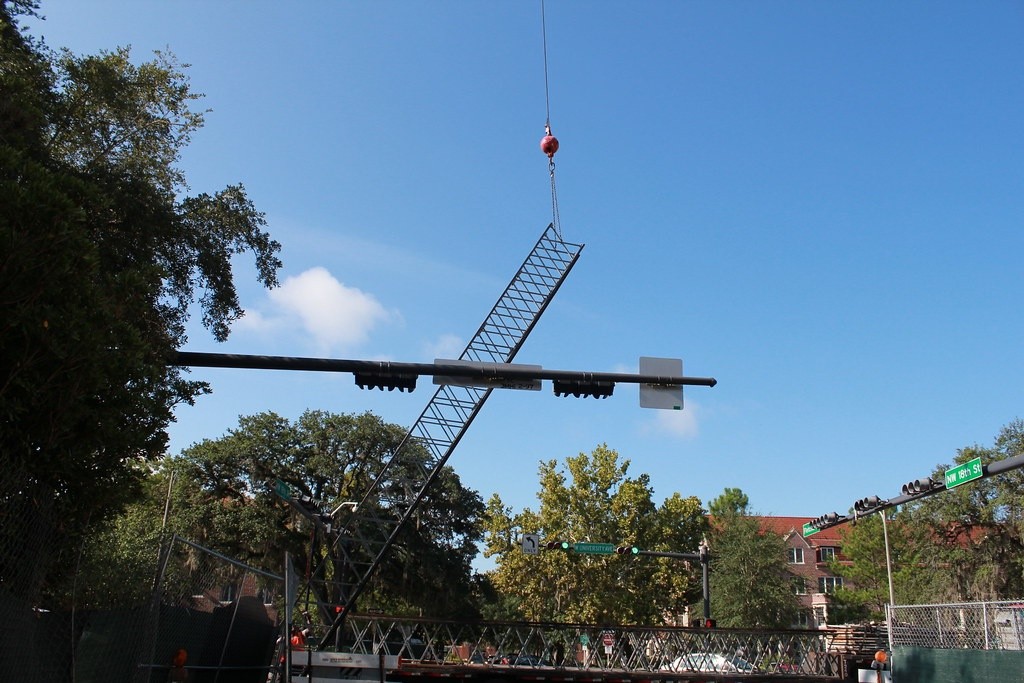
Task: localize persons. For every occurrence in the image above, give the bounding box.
[276,611,314,683]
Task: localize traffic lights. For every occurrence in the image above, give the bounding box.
[616,546,640,554]
[333,604,342,613]
[901,478,932,495]
[855,495,877,511]
[705,618,716,629]
[546,541,569,549]
[810,512,836,529]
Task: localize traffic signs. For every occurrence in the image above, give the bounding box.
[944,457,982,488]
[573,542,614,554]
[802,522,821,537]
[276,479,291,500]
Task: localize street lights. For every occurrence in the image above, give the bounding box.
[304,502,360,616]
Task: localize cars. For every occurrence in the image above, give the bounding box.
[661,652,757,675]
[490,654,551,667]
[379,637,436,659]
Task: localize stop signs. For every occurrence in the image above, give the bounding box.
[604,633,615,647]
